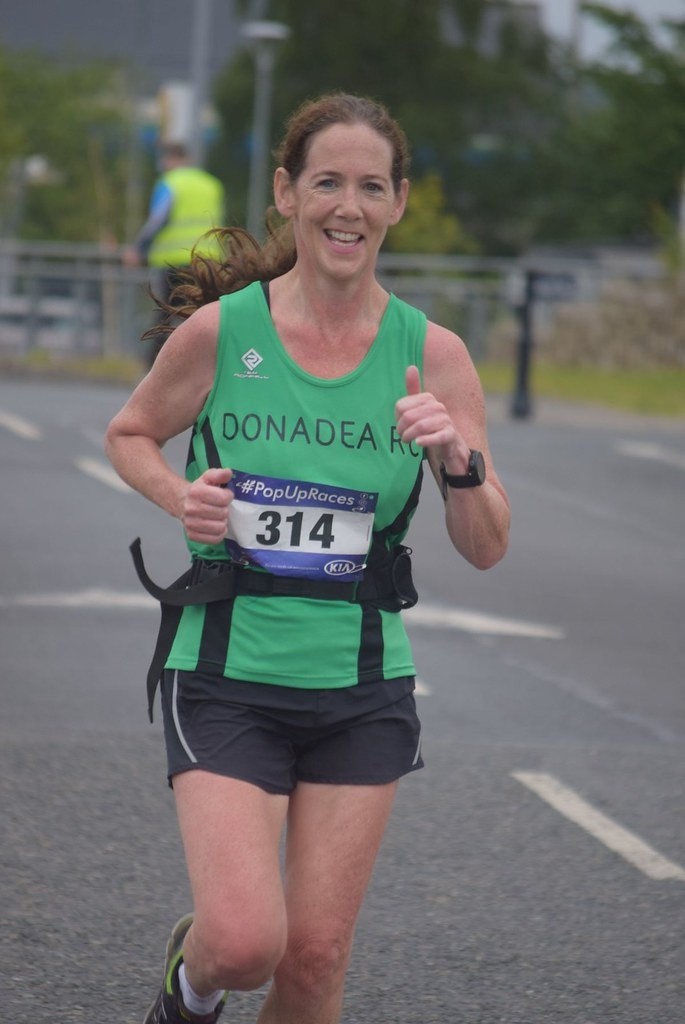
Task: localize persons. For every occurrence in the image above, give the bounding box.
[106,97,514,1024]
[135,137,237,374]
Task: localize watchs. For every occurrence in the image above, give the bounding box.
[439,447,486,501]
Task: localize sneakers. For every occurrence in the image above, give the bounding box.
[142,912,231,1024]
